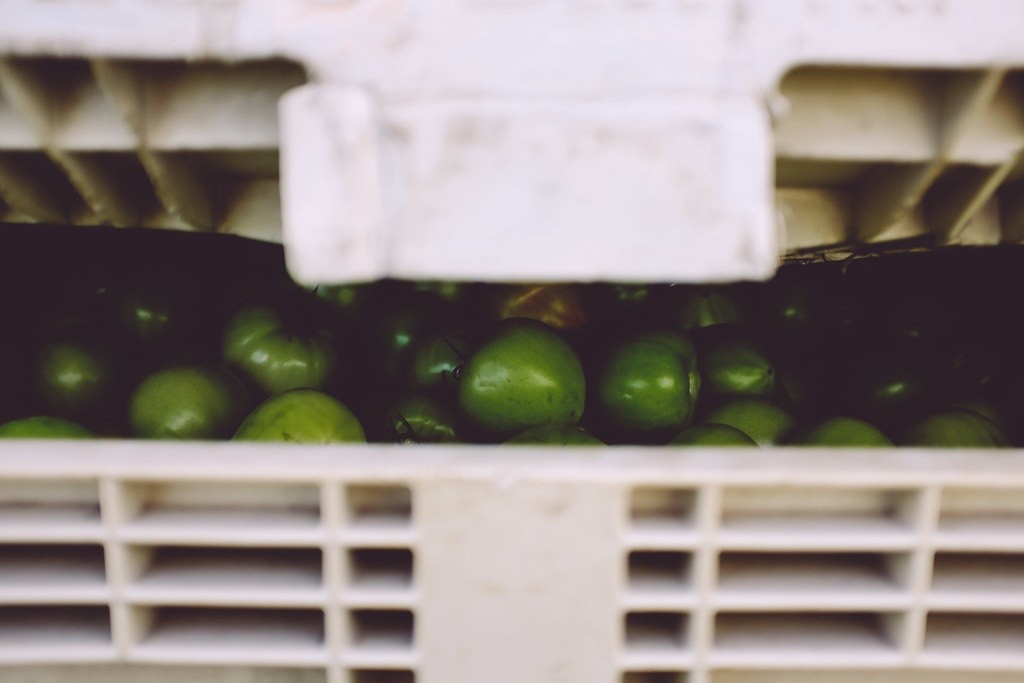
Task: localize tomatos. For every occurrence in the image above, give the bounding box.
[0,245,1024,451]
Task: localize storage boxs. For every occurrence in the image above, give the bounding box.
[0,0,1024,683]
